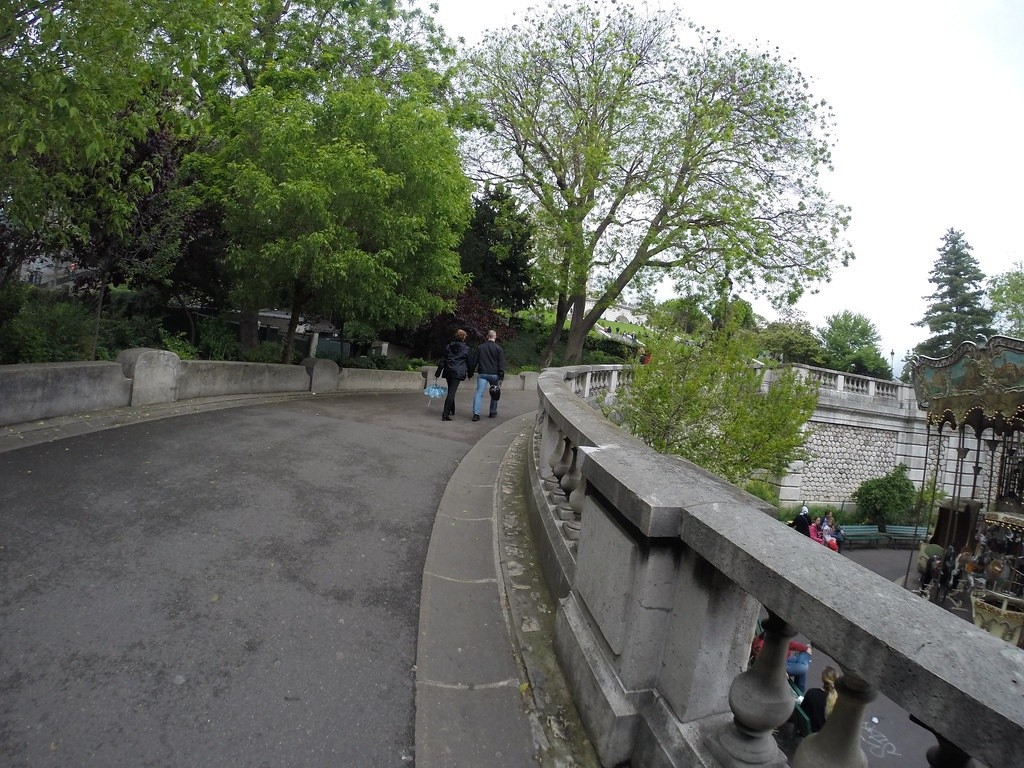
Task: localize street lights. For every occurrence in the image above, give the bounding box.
[890,348,895,373]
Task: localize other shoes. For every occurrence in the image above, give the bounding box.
[472,413,481,421]
[489,413,497,418]
[442,410,456,419]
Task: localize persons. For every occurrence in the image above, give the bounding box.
[638,353,645,365]
[799,666,838,733]
[469,331,504,421]
[787,506,846,553]
[631,332,637,341]
[435,328,470,421]
[605,326,612,333]
[752,618,811,692]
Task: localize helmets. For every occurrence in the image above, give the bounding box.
[489,384,501,402]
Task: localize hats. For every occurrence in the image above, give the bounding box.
[800,506,809,516]
[961,547,973,556]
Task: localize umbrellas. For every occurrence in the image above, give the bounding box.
[423,378,448,408]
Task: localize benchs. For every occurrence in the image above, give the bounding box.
[885,525,932,551]
[788,676,812,738]
[840,525,889,551]
